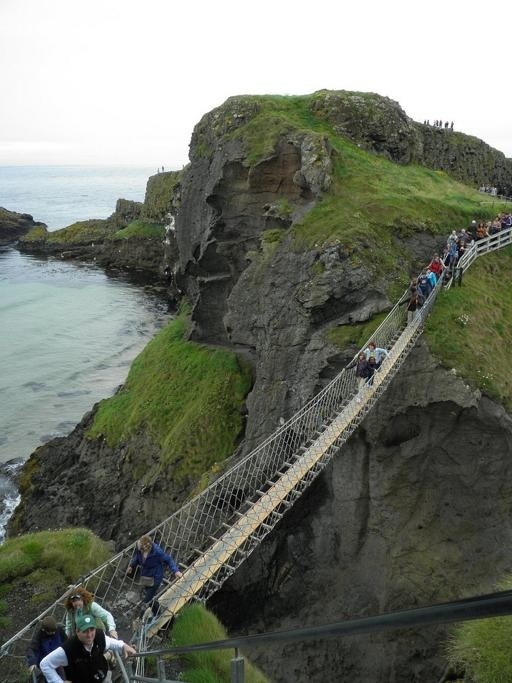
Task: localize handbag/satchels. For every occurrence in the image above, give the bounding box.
[139,576,155,586]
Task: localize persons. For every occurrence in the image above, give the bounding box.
[25,615,69,682]
[127,536,183,616]
[366,356,382,388]
[423,119,454,129]
[363,341,390,384]
[397,212,511,327]
[63,586,120,639]
[40,614,137,682]
[343,351,377,402]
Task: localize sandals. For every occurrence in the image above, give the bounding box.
[108,650,117,670]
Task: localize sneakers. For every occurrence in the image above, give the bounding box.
[152,612,160,619]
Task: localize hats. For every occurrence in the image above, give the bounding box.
[36,616,58,631]
[77,615,97,632]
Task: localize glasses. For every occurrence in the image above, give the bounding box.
[71,595,80,599]
